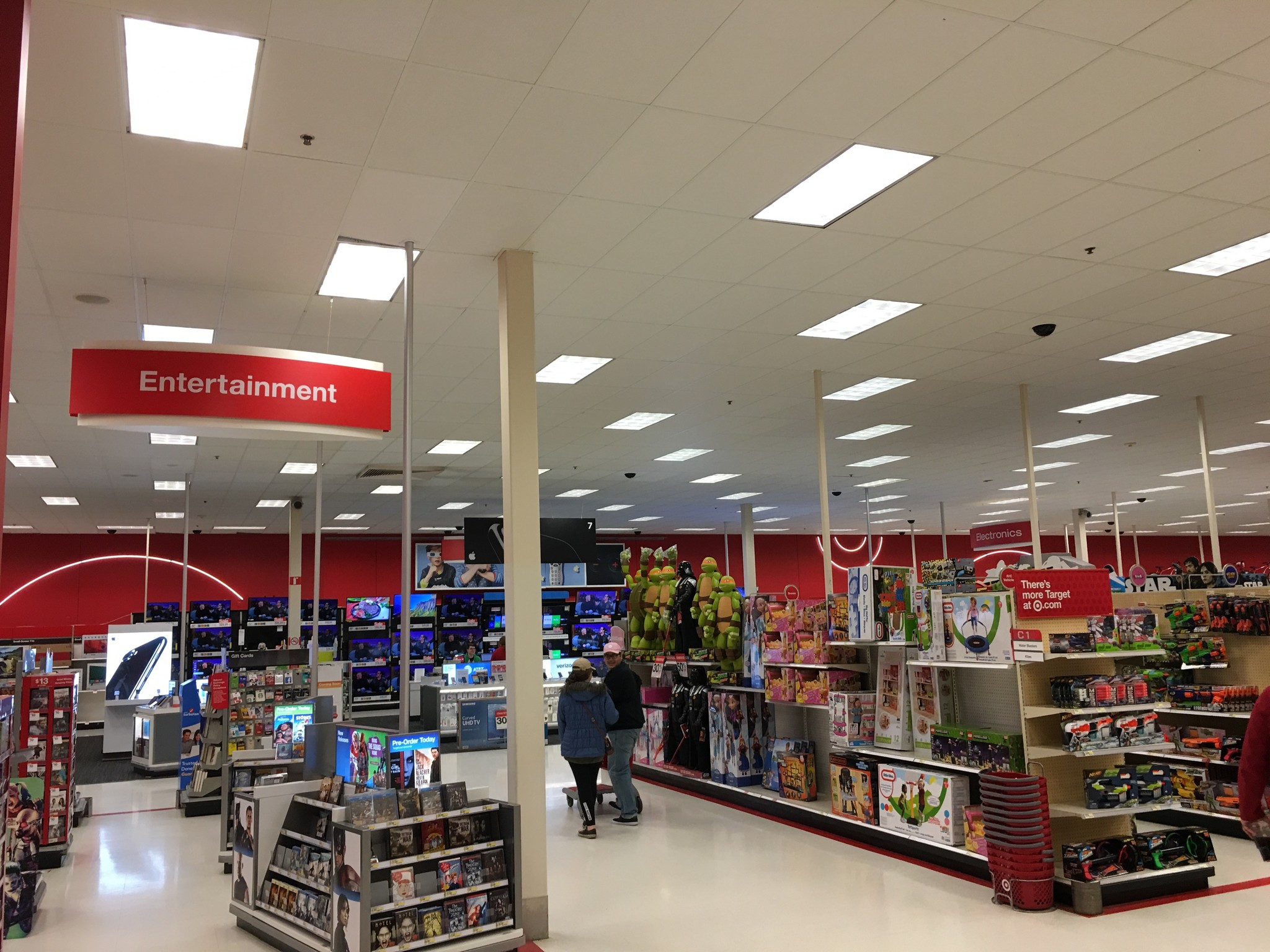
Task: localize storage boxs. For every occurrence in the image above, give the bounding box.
[848,565,918,642]
[920,557,976,594]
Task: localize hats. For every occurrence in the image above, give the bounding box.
[572,658,596,671]
[603,642,621,654]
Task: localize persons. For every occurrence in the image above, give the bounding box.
[463,633,480,654]
[576,628,594,650]
[224,733,518,952]
[444,634,463,657]
[742,597,769,641]
[591,627,611,649]
[464,643,481,663]
[1171,556,1219,591]
[710,692,743,741]
[144,596,436,754]
[417,545,456,588]
[3,712,69,914]
[490,636,506,661]
[602,642,645,826]
[446,597,480,619]
[557,657,619,838]
[595,594,613,614]
[578,594,596,615]
[453,562,505,588]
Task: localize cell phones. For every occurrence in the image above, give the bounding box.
[487,523,582,563]
[106,637,167,699]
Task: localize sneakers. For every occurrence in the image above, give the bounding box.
[582,821,587,829]
[578,827,597,838]
[612,816,638,826]
[608,801,622,812]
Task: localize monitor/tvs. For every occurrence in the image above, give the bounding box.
[144,588,632,712]
[83,639,107,655]
[89,665,106,685]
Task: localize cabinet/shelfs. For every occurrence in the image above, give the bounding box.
[0,584,1270,952]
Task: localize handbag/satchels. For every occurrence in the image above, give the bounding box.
[604,739,613,751]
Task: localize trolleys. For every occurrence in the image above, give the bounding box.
[562,751,642,814]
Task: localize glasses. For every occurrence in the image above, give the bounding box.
[426,552,441,557]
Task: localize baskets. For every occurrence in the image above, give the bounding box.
[978,767,1055,910]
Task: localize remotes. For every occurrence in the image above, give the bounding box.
[549,562,563,586]
